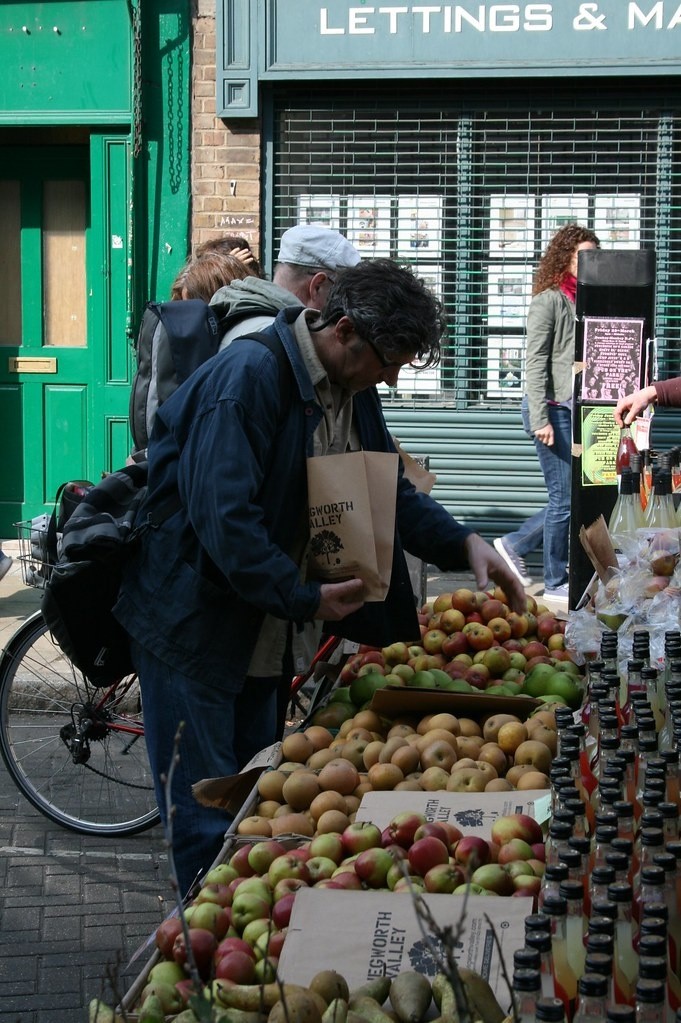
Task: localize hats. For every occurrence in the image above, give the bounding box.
[276,221,361,272]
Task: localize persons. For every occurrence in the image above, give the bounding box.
[126,237,265,466]
[612,377,681,428]
[208,223,359,355]
[141,262,528,901]
[493,225,600,602]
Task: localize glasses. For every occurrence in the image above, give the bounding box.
[358,329,403,369]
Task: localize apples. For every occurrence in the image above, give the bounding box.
[143,812,546,1002]
[310,584,583,727]
[582,531,681,631]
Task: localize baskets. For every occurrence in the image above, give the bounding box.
[14,514,65,589]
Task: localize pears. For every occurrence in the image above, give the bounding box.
[69,968,520,1022]
[233,704,562,834]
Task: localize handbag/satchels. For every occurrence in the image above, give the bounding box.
[38,459,146,689]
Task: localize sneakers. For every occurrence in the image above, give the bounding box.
[543,582,569,604]
[494,536,533,588]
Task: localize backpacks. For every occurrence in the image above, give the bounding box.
[128,301,278,463]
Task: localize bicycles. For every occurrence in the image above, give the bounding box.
[0,455,447,839]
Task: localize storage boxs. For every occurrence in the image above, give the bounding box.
[116,674,563,1019]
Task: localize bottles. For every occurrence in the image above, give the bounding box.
[607,443,681,535]
[514,629,681,1023]
[615,410,641,495]
